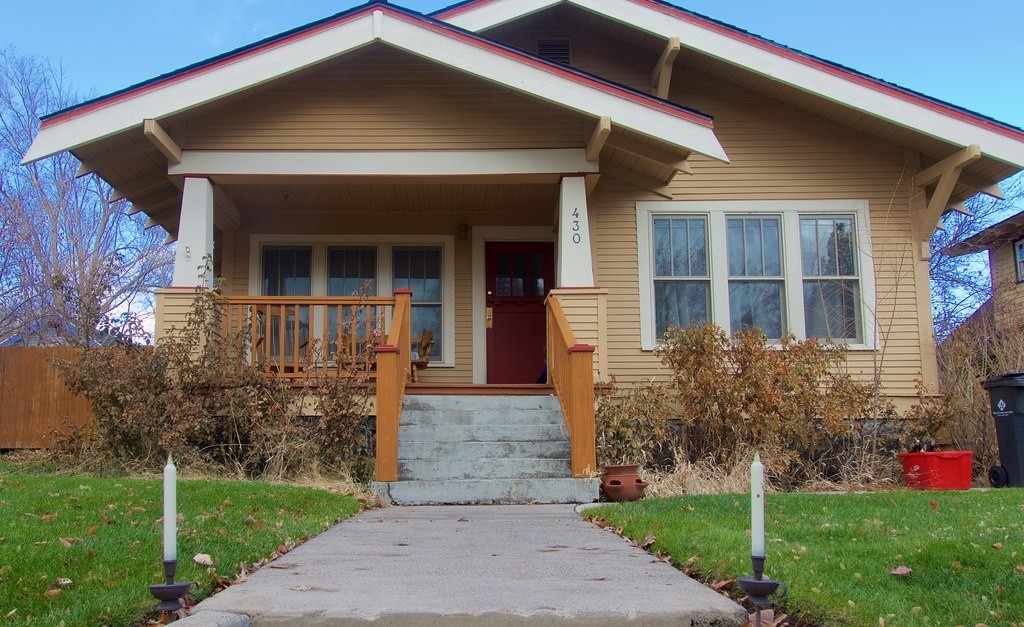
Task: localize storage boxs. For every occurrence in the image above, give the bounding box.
[895,451,974,490]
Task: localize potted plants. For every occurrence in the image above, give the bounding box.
[596,377,674,502]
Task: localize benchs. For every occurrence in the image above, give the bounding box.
[336,328,434,383]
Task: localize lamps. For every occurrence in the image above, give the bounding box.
[735,452,787,606]
[149,452,199,610]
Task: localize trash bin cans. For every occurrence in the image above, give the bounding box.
[980,372,1024,488]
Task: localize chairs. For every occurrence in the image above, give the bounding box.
[248,304,307,382]
